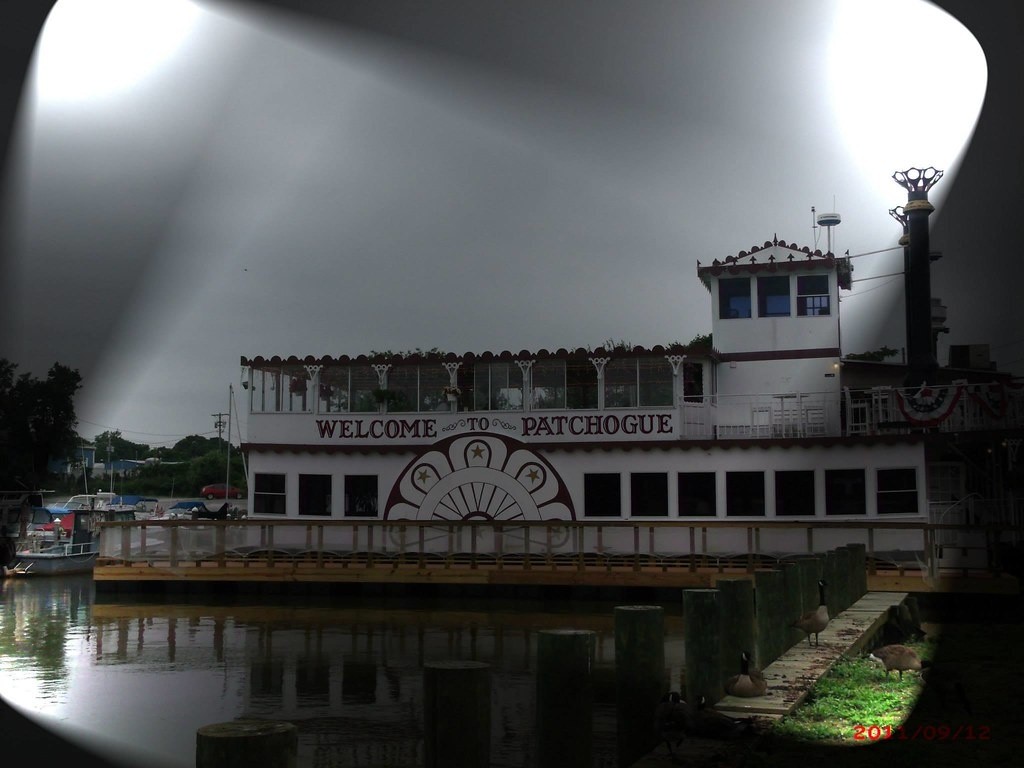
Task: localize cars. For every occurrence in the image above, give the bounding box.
[202,483,246,500]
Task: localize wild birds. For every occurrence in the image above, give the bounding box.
[870,643,933,684]
[787,580,830,649]
[652,694,696,753]
[724,650,767,697]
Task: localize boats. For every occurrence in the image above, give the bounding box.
[0,494,208,577]
